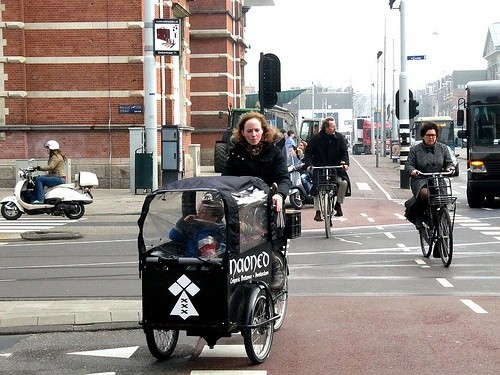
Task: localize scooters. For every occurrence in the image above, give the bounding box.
[283,162,317,208]
[0,170,99,219]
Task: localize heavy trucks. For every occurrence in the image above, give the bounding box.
[352,119,391,155]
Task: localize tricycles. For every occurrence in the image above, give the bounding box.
[137,176,287,364]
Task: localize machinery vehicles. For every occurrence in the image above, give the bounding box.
[213,107,325,172]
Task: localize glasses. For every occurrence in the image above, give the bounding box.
[425,134,436,136]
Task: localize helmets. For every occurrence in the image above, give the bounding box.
[44,140,59,150]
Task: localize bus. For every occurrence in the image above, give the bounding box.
[411,116,456,149]
[457,79,500,207]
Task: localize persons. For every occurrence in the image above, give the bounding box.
[304,117,349,220]
[32,139,68,204]
[222,110,291,292]
[281,129,311,197]
[170,200,226,257]
[404,121,457,258]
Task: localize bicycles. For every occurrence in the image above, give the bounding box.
[408,169,456,267]
[308,165,349,238]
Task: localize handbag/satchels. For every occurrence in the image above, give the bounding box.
[404,196,424,225]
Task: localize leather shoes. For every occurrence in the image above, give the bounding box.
[269,258,285,289]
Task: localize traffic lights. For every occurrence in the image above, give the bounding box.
[409,91,419,120]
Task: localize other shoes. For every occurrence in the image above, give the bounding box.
[31,200,43,203]
[334,203,343,216]
[314,210,321,220]
[416,217,424,229]
